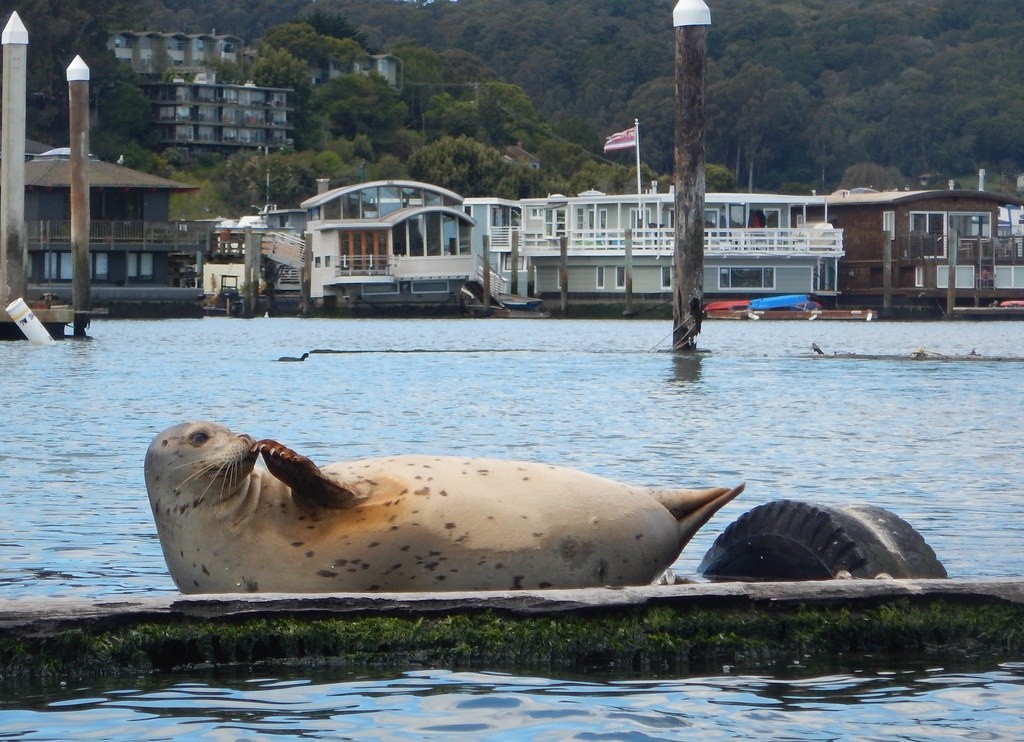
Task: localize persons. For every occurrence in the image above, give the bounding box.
[749,209,767,228]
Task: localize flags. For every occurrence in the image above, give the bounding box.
[603,127,637,154]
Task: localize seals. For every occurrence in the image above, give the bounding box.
[144,421,745,594]
